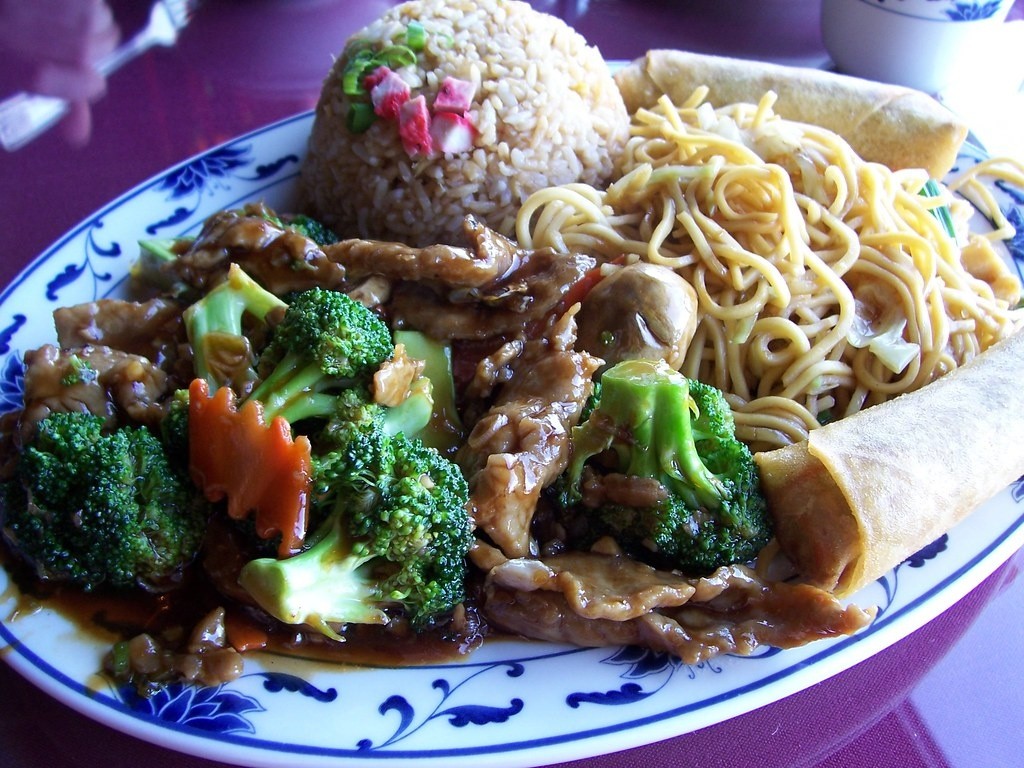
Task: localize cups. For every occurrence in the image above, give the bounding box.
[819,0,1017,97]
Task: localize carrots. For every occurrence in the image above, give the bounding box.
[190,379,311,554]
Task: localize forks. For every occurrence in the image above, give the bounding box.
[0,0,195,154]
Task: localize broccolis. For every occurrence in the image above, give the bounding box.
[238,288,392,422]
[548,359,770,565]
[1,407,202,586]
[240,436,473,633]
[161,264,284,443]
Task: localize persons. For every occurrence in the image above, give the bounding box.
[0,0,116,151]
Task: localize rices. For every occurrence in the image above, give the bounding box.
[302,1,637,244]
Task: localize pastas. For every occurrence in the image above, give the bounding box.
[512,86,1024,456]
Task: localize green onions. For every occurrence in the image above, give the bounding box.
[341,21,453,129]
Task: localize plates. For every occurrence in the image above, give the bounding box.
[0,60,1024,768]
